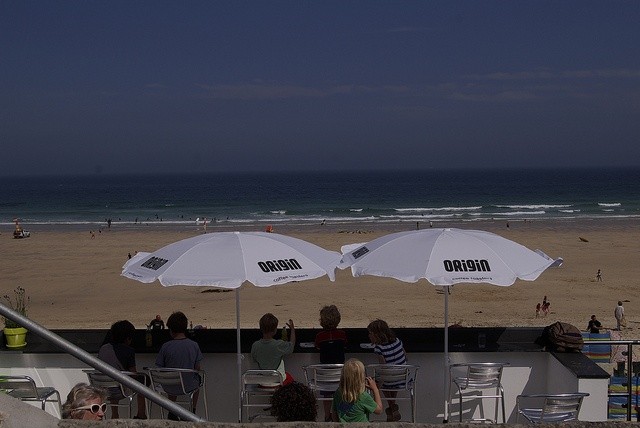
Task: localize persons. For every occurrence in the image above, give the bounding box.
[368,319,407,421]
[315,305,348,420]
[269,379,321,422]
[60,383,108,420]
[88,212,231,239]
[101,320,151,418]
[332,359,383,422]
[156,312,204,421]
[614,301,625,331]
[251,313,297,389]
[536,296,550,318]
[586,315,603,334]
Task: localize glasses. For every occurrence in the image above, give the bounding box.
[71,402,108,415]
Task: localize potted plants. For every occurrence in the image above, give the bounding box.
[5,290,28,348]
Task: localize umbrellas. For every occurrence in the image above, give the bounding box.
[341,226,562,340]
[122,230,341,356]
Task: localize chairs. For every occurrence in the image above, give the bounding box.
[3,375,65,419]
[238,367,285,423]
[148,357,209,420]
[373,365,420,423]
[449,362,508,427]
[77,360,147,417]
[305,361,347,420]
[516,391,590,427]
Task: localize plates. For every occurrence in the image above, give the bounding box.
[300,342,316,349]
[360,343,374,348]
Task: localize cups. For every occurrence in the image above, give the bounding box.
[479,334,486,348]
